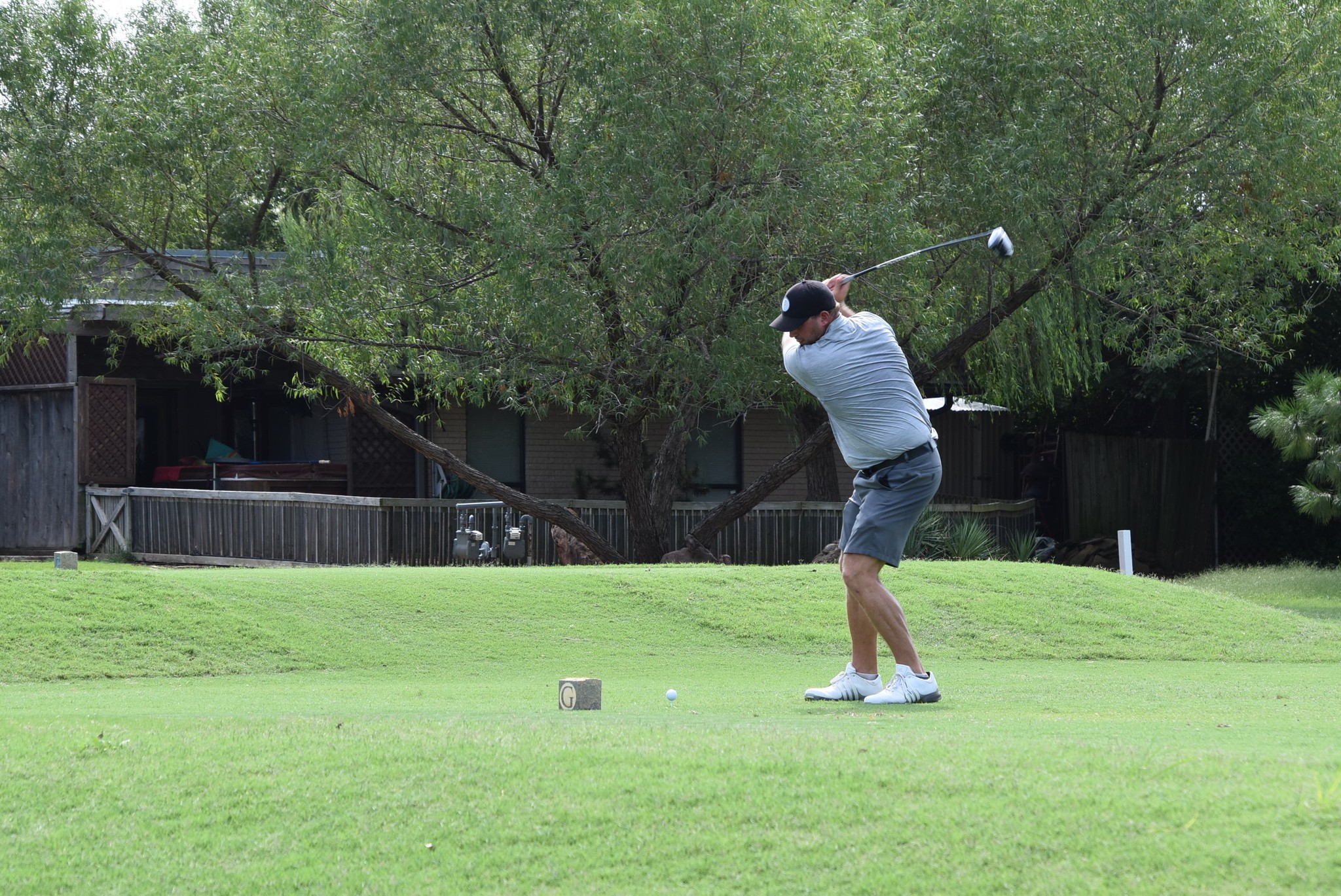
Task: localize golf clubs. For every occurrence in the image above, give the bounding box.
[840,227,1014,287]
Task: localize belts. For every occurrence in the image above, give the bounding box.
[865,438,938,474]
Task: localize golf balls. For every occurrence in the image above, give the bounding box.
[666,689,677,701]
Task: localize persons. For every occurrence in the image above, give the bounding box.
[767,274,942,705]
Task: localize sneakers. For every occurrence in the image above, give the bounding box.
[805,662,883,701]
[864,663,942,705]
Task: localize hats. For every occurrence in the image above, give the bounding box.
[769,280,835,332]
